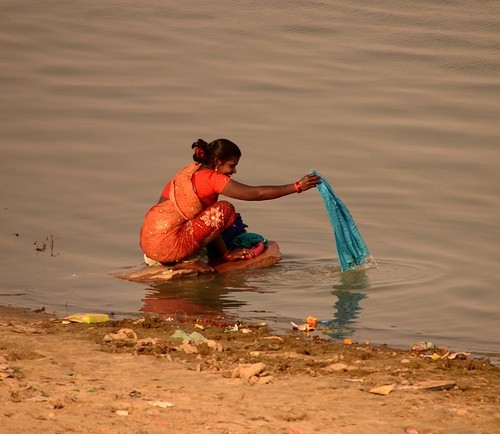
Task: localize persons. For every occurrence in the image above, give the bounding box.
[138,137,323,275]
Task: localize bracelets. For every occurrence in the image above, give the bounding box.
[294,181,302,194]
